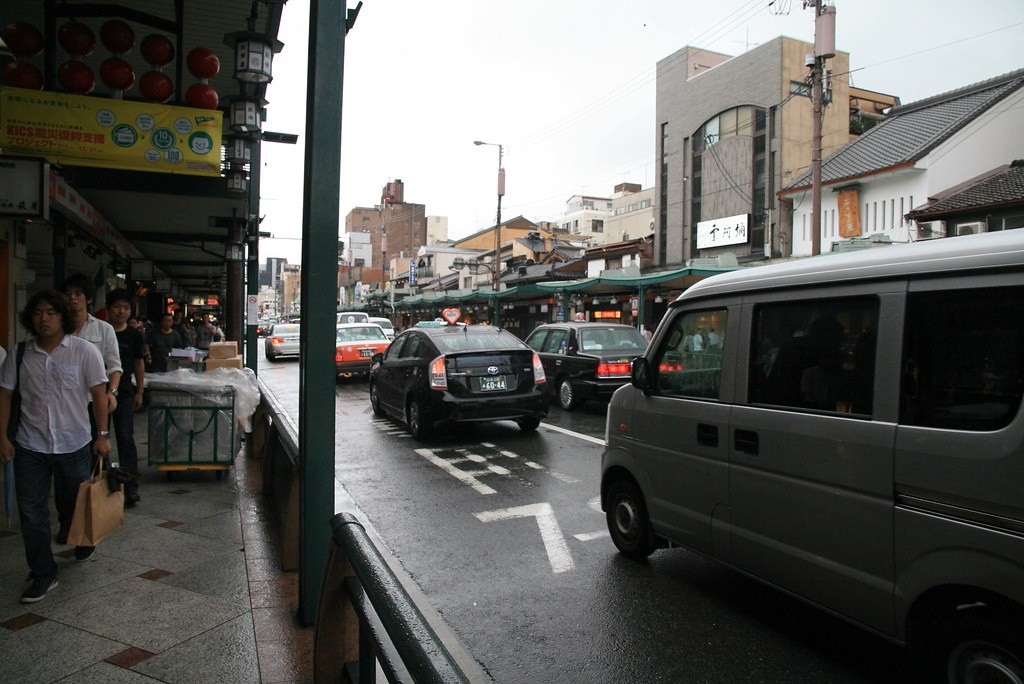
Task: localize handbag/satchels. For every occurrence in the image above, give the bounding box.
[6,342,26,438]
[66,455,125,546]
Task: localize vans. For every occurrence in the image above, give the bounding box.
[598,225,1024,684]
[336,311,370,335]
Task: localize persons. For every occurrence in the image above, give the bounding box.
[127,307,225,373]
[56,272,123,544]
[0,290,113,602]
[104,287,145,504]
[641,325,653,344]
[684,326,719,365]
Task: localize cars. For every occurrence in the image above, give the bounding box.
[369,316,396,340]
[264,323,300,362]
[257,318,300,338]
[335,317,393,381]
[523,312,650,414]
[369,307,548,442]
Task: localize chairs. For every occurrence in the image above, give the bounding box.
[360,329,369,337]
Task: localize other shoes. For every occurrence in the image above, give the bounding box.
[55,518,72,544]
[126,491,141,502]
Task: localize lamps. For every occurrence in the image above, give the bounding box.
[611,293,618,304]
[68,233,126,279]
[592,294,599,304]
[655,292,662,303]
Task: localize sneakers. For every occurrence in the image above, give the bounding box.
[20,574,58,602]
[75,547,95,561]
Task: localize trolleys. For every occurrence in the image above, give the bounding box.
[144,380,248,482]
[164,345,209,374]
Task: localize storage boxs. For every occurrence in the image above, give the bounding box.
[206,341,243,371]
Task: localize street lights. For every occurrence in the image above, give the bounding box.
[472,140,505,327]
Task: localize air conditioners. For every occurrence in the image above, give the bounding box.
[957,221,985,237]
[649,218,655,235]
[916,220,946,241]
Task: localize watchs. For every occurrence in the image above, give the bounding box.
[109,388,118,397]
[98,431,109,438]
